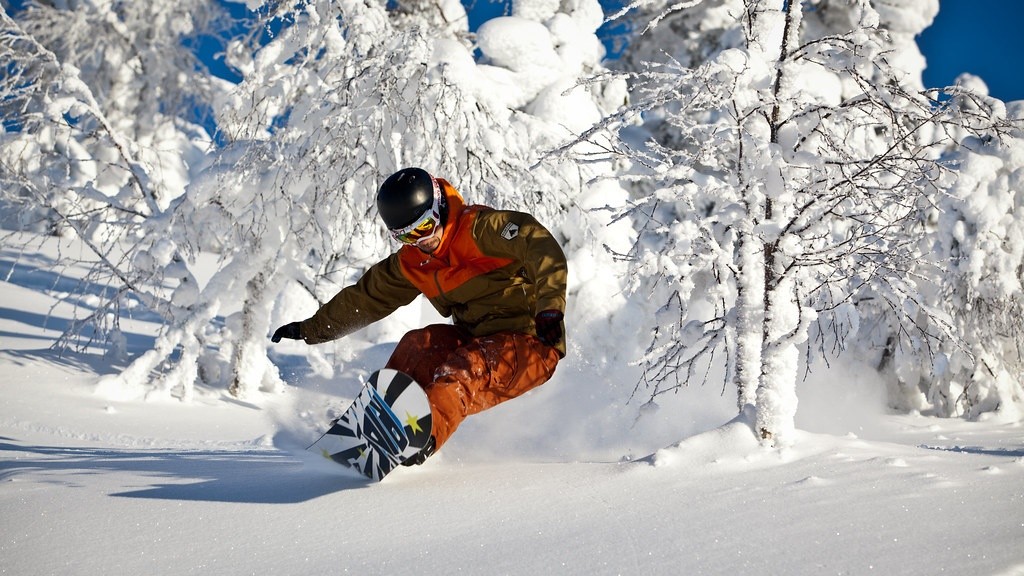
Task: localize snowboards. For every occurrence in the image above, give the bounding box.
[305,367,433,483]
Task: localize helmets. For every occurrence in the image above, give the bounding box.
[376,167,448,230]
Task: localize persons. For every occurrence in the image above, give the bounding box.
[268,168,569,467]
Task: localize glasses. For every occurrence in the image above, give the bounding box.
[391,209,440,245]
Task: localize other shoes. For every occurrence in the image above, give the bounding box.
[399,441,434,467]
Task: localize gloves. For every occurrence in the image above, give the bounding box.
[272,321,302,343]
[535,310,563,346]
